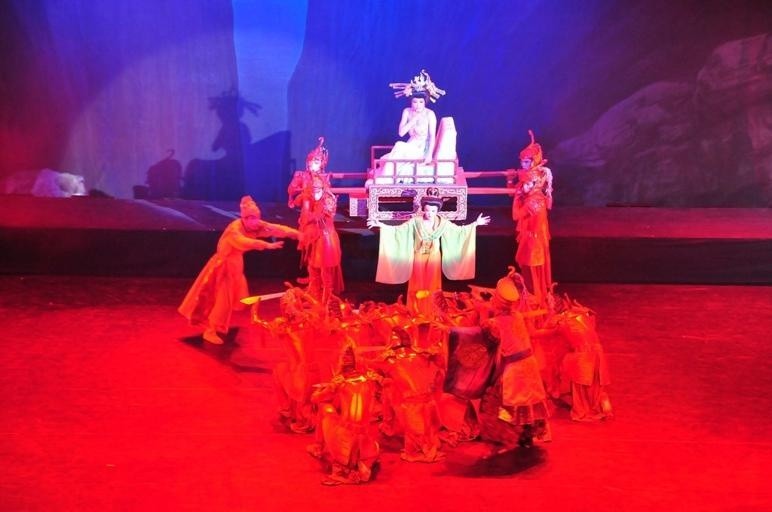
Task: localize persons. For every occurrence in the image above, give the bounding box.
[243,265,611,485]
[506,144,553,242]
[364,91,436,185]
[178,195,304,344]
[366,187,491,304]
[505,171,553,298]
[294,177,345,293]
[288,146,329,285]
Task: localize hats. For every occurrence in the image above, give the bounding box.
[525,170,546,195]
[520,144,542,169]
[493,278,519,307]
[305,146,328,173]
[307,175,325,202]
[240,196,261,217]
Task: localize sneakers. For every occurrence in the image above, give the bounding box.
[203,326,223,345]
